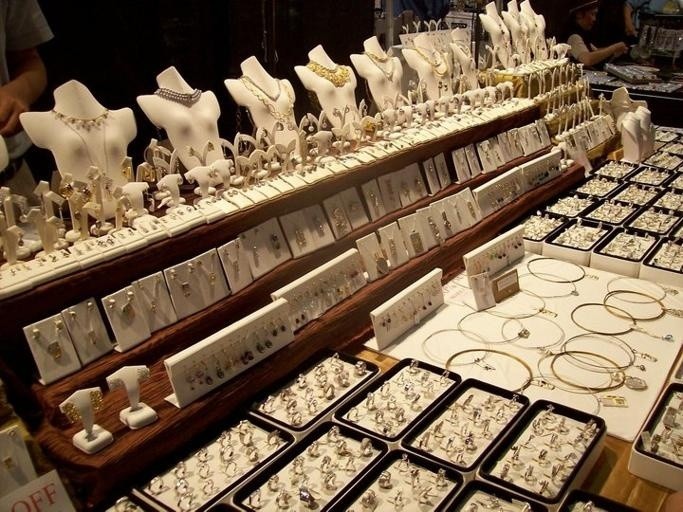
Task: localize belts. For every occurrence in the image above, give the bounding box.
[0,158,23,185]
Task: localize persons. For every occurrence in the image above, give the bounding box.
[561,0,629,73]
[0,0,56,225]
[623,0,672,46]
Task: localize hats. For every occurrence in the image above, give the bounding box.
[569,1,598,16]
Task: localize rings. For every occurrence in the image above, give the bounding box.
[520,128,682,271]
[101,347,607,512]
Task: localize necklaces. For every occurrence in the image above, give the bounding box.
[0,13,546,359]
